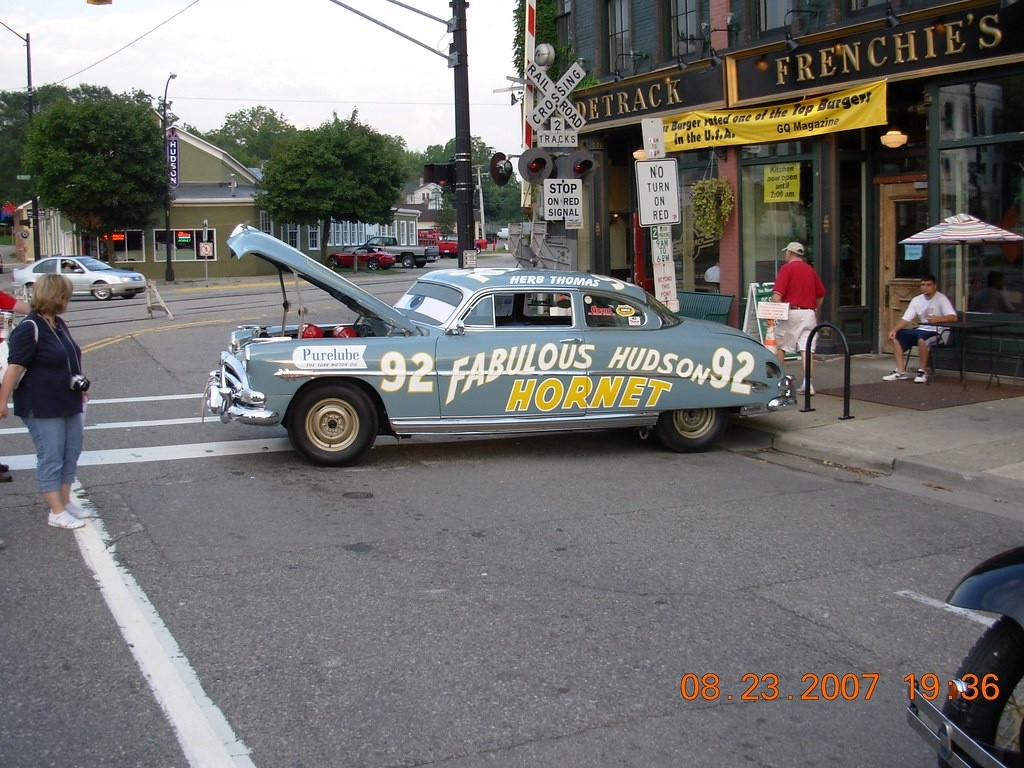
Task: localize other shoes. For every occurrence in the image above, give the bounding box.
[0,465,8,472]
[795,384,815,395]
[914,369,927,382]
[882,368,908,381]
[0,473,12,482]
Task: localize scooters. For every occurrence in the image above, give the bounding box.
[905,545,1024,768]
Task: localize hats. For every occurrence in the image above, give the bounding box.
[782,242,804,255]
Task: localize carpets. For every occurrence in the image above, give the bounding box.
[815,376,1024,412]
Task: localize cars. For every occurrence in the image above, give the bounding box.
[0,255,3,274]
[435,234,488,259]
[206,221,798,468]
[11,255,146,301]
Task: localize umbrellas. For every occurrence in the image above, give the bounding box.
[899,211,1024,383]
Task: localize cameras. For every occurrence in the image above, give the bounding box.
[71,375,90,391]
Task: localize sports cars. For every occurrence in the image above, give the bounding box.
[326,245,396,270]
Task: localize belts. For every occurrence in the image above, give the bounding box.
[791,307,815,309]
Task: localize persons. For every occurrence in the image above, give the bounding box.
[0,272,93,549]
[703,260,720,284]
[881,273,958,384]
[769,242,826,396]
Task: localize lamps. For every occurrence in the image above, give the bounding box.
[783,9,822,53]
[708,24,740,67]
[676,34,710,71]
[880,121,908,150]
[613,51,648,83]
[883,0,901,31]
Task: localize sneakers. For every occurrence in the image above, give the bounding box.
[64,501,91,518]
[48,509,85,529]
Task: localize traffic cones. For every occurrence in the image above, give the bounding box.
[763,320,779,356]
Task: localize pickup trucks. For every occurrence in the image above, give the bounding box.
[353,235,441,269]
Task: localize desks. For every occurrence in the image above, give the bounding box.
[917,320,1024,390]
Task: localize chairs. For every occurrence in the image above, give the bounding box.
[905,326,962,383]
[986,335,1024,399]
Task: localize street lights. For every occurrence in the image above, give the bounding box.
[162,73,176,282]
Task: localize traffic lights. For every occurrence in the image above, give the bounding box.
[563,150,597,181]
[423,162,456,186]
[517,148,552,184]
[489,152,513,187]
